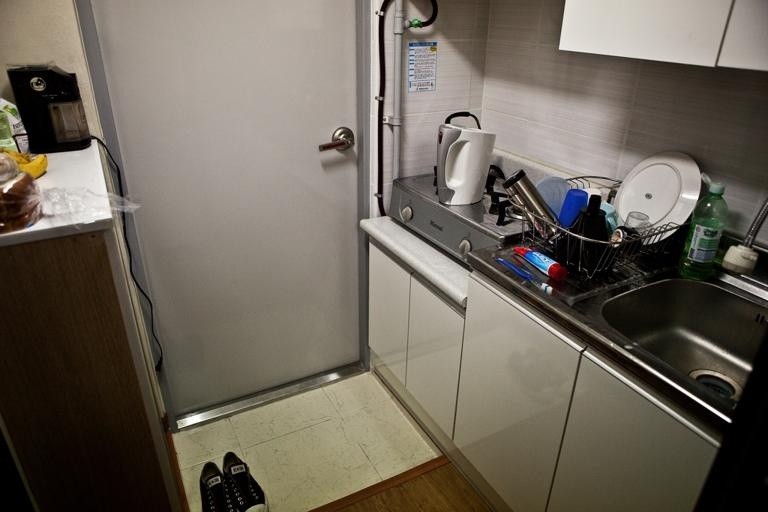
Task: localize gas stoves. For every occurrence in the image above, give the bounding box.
[388,162,524,265]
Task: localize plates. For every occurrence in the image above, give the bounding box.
[613,152,703,246]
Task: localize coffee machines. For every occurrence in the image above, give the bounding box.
[5,61,92,152]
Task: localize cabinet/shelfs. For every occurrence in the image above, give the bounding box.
[361,236,465,441]
[0,141,184,512]
[452,271,723,511]
[558,1,767,73]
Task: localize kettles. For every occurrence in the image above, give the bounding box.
[435,122,498,205]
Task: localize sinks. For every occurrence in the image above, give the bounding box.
[572,270,768,404]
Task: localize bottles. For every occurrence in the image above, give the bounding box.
[680,171,729,281]
[503,168,561,241]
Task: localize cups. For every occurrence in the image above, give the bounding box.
[558,186,588,228]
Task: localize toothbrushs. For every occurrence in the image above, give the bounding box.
[496,257,554,296]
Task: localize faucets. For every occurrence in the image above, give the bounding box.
[722,196,768,268]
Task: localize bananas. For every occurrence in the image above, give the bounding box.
[0,146,48,180]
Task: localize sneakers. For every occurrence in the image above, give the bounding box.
[199,452,267,512]
